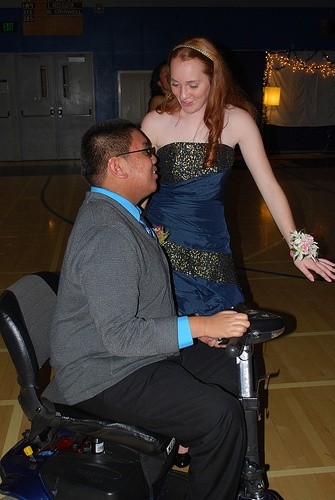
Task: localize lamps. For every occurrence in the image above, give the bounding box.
[263,86,281,106]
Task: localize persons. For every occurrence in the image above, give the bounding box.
[41,118,251,500]
[138,35,335,468]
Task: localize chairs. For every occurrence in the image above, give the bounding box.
[0,271,180,500]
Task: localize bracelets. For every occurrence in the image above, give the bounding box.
[290,230,319,262]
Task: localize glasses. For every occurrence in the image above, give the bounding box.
[113,146,157,158]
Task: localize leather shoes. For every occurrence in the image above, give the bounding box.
[174,452,192,468]
